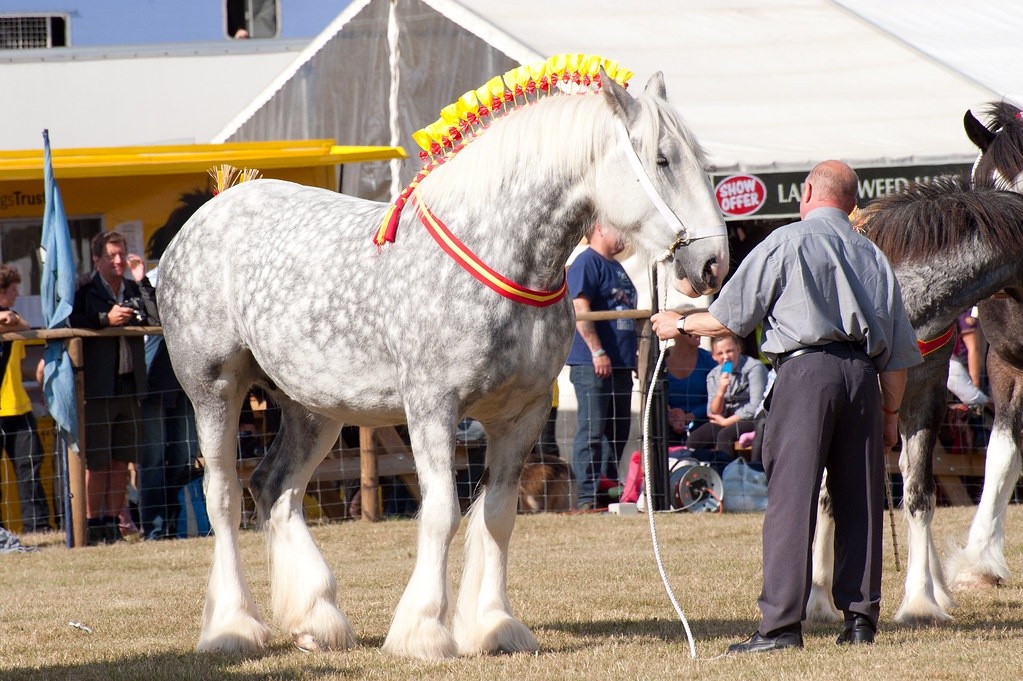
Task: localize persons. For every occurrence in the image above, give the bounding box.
[650,160,924,652]
[0,261,51,533]
[566,225,639,510]
[69,231,159,544]
[652,303,715,449]
[932,308,993,449]
[135,332,188,540]
[177,378,578,537]
[685,332,768,474]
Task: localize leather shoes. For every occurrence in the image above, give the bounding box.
[727,623,803,653]
[836,611,877,648]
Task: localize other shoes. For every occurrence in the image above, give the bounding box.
[22,526,56,534]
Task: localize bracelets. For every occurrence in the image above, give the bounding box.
[593,349,605,356]
[881,406,900,415]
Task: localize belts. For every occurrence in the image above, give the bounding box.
[775,340,865,366]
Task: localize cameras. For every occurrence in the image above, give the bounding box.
[120,297,149,325]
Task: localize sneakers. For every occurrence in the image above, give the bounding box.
[576,501,608,512]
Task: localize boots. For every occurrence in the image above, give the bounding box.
[87,516,102,546]
[106,515,127,545]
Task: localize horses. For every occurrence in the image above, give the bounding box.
[803,100,1023,624]
[154,66,731,659]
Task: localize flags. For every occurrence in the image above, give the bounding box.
[39,144,81,456]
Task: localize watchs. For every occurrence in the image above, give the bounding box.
[678,316,687,335]
